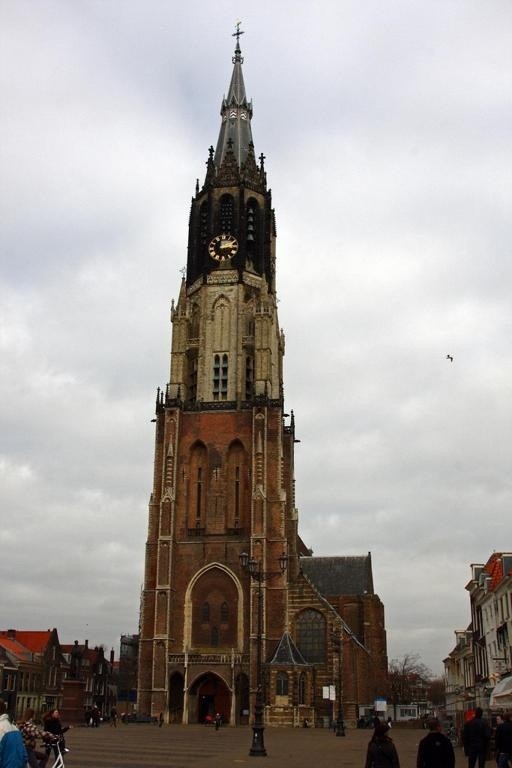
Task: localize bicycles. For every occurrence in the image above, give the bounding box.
[26,738,68,768]
[448,727,458,748]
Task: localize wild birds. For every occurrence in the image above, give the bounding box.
[446,355,453,362]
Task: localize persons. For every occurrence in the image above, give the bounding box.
[0,697,74,768]
[493,713,512,768]
[416,718,455,768]
[84,704,164,728]
[302,715,393,733]
[203,710,226,731]
[408,709,456,737]
[364,724,400,768]
[461,707,491,768]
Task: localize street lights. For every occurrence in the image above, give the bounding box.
[238,553,287,756]
[330,628,354,736]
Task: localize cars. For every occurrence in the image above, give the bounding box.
[123,711,157,723]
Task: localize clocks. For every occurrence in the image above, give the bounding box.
[209,235,239,261]
